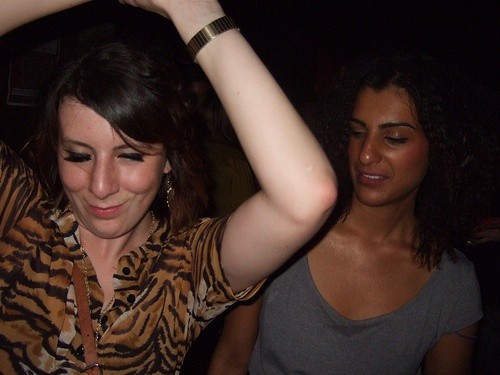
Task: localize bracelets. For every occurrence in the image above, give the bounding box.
[188,14,243,62]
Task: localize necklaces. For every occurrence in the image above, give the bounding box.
[77,213,158,349]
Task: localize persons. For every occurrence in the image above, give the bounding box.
[0,0,337,375]
[208,68,483,375]
